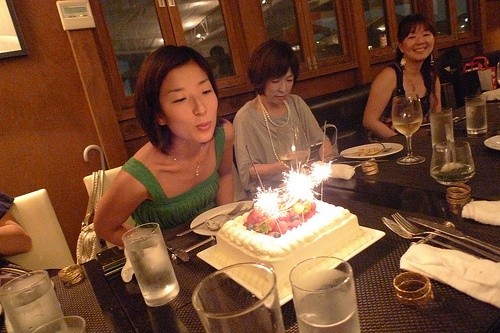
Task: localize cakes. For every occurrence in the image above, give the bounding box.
[216,190,361,294]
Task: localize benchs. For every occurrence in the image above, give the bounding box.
[306,49,500,152]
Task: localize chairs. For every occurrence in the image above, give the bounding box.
[4,188,76,270]
[83,165,139,248]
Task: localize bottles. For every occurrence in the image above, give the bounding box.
[0,267,86,333]
[379,31,387,46]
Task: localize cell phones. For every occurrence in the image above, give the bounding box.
[96,246,126,272]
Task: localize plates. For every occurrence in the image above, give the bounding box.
[190,199,258,237]
[340,142,404,158]
[484,135,500,151]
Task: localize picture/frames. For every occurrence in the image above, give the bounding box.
[0,0,31,61]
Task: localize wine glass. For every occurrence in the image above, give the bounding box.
[391,93,426,166]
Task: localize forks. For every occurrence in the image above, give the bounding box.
[176,203,244,237]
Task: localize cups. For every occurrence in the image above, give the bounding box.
[429,139,477,188]
[289,255,362,333]
[465,95,488,134]
[121,223,180,307]
[192,263,287,333]
[269,119,310,168]
[429,106,453,151]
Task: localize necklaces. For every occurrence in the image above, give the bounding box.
[169,145,206,176]
[258,94,294,161]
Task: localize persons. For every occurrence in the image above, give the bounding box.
[362,13,442,139]
[211,46,231,77]
[232,40,335,196]
[93,45,235,248]
[0,194,33,268]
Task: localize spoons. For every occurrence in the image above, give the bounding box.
[381,215,466,251]
[391,212,500,262]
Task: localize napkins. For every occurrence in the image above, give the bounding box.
[460,199,500,225]
[398,243,500,308]
[329,164,355,180]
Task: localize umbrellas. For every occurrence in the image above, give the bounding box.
[83,145,106,171]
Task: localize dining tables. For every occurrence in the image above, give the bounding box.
[0,100,500,333]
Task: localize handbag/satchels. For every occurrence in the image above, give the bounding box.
[441,70,482,109]
[463,56,496,90]
[76,170,108,265]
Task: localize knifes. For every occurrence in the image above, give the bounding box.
[166,244,190,262]
[408,217,500,253]
